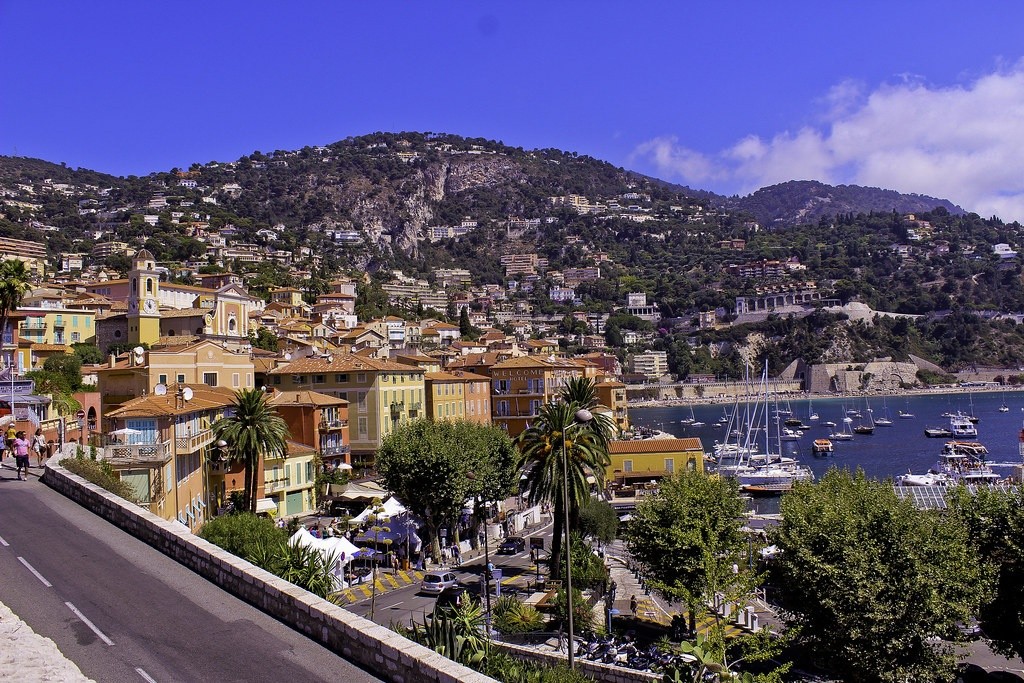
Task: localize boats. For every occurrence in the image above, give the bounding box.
[893,411,1000,485]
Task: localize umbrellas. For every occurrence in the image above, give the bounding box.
[108,427,141,445]
[338,463,353,474]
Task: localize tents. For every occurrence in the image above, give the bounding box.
[287,496,409,594]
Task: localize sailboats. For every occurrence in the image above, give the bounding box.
[813,384,918,458]
[681,399,707,427]
[999,389,1010,412]
[713,355,820,496]
[968,392,979,424]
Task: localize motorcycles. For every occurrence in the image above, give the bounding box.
[574,630,718,683]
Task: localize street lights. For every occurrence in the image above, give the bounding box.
[560,408,594,673]
[466,470,492,641]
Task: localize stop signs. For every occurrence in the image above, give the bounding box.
[77,409,85,419]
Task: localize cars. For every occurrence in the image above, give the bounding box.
[497,537,525,555]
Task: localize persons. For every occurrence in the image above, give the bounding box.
[0,423,50,480]
[390,521,535,575]
[278,513,336,539]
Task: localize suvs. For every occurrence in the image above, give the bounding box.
[420,572,457,596]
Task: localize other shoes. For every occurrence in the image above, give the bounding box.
[19,475,27,481]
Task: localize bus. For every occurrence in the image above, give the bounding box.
[929,381,1001,387]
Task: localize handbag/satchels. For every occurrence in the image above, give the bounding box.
[39,446,46,453]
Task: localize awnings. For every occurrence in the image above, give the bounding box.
[179,499,206,525]
